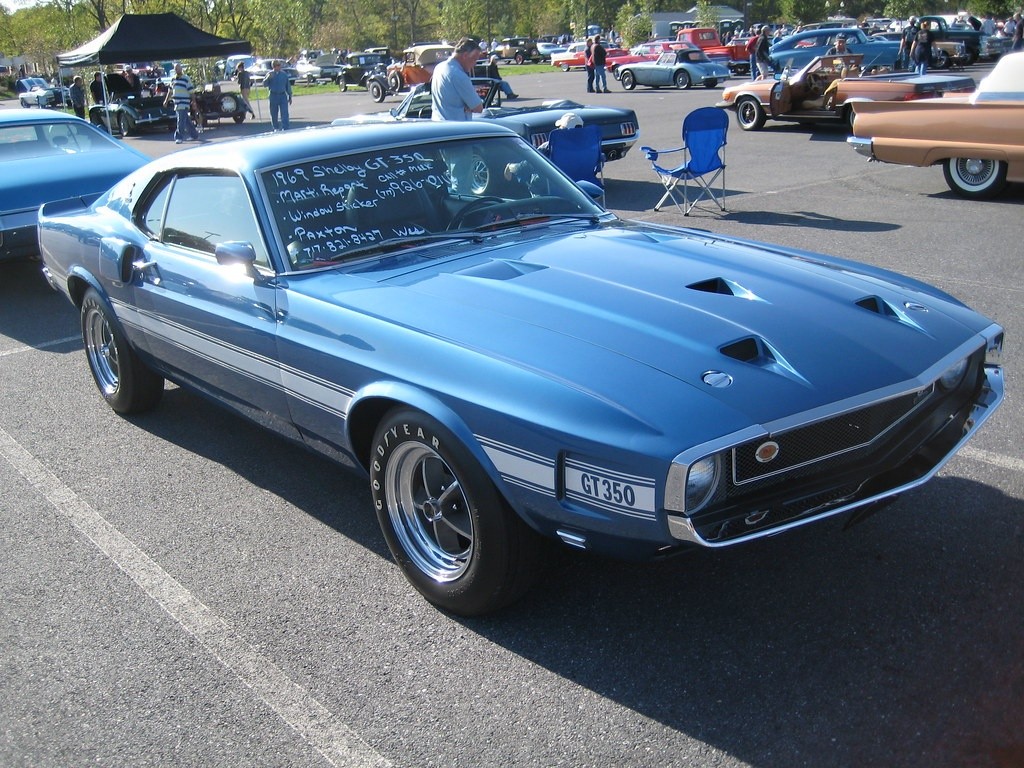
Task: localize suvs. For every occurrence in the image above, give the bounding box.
[104,64,166,88]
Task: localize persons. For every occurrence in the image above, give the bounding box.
[262,59,292,131]
[162,63,199,144]
[721,23,803,47]
[478,25,621,93]
[430,36,485,198]
[89,71,107,105]
[236,61,256,120]
[825,32,851,55]
[504,112,584,199]
[898,16,918,69]
[214,63,219,75]
[872,21,877,27]
[487,53,519,99]
[951,12,1024,57]
[857,17,870,36]
[68,75,85,120]
[745,25,761,81]
[755,25,779,79]
[124,65,141,97]
[674,26,683,42]
[909,19,941,75]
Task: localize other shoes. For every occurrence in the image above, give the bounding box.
[596,90,603,93]
[507,94,519,99]
[587,89,595,92]
[603,90,612,93]
[250,115,255,119]
[175,138,182,143]
[187,137,194,141]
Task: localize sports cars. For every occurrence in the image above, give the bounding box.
[613,48,731,92]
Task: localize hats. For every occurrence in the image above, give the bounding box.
[491,55,500,61]
[836,33,847,40]
[908,16,917,21]
[272,60,281,67]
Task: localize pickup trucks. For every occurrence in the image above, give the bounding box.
[673,28,753,76]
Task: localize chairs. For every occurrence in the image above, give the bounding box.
[516,124,606,210]
[869,68,888,75]
[816,36,825,47]
[841,66,859,80]
[638,106,730,217]
[345,165,439,233]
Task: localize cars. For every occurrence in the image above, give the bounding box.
[35,119,1009,617]
[0,105,158,264]
[0,65,11,77]
[329,77,643,196]
[366,45,491,103]
[845,53,1024,198]
[714,54,978,136]
[90,73,179,136]
[770,16,1015,73]
[189,88,251,126]
[606,41,729,81]
[19,79,69,110]
[216,47,402,92]
[161,65,198,87]
[489,26,628,73]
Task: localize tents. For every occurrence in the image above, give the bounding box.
[56,11,262,137]
